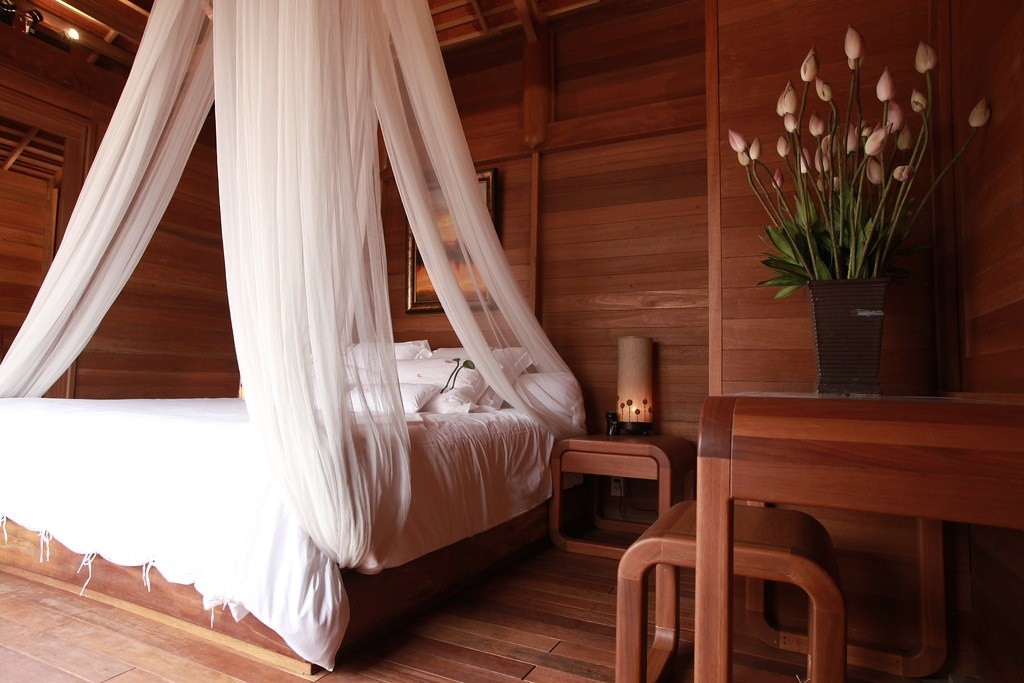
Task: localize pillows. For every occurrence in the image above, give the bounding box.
[341,380,441,415]
[340,340,431,372]
[396,357,502,416]
[430,347,535,409]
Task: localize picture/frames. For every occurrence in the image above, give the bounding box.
[404,167,503,315]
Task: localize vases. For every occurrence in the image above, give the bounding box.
[801,277,893,398]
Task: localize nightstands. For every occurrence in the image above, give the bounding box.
[546,432,696,561]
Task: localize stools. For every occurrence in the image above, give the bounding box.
[613,501,849,683]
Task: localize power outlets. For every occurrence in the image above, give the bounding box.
[610,476,625,497]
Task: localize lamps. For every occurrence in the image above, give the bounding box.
[602,335,657,437]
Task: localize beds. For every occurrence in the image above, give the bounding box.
[0,369,592,677]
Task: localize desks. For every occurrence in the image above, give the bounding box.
[693,388,1023,683]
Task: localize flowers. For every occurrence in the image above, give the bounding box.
[726,19,994,300]
